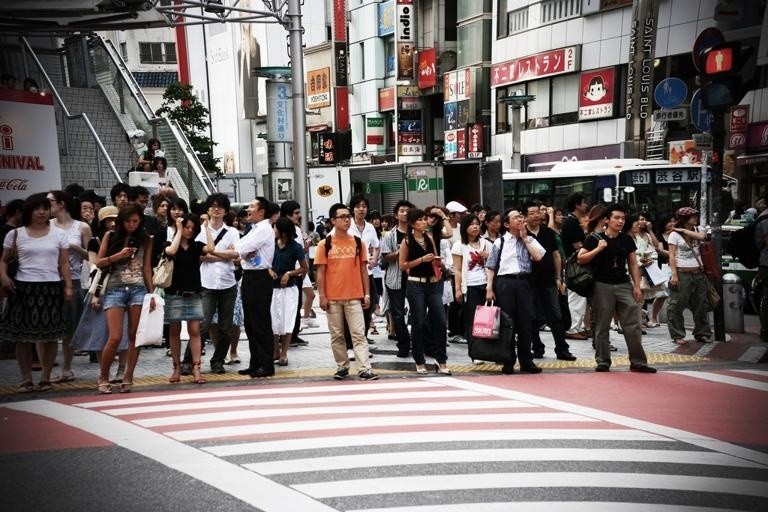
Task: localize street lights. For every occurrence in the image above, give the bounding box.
[495,89,536,170]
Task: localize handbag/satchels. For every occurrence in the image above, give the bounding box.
[79,236,102,290]
[563,231,602,299]
[640,273,664,291]
[152,246,174,289]
[269,273,294,289]
[428,233,443,284]
[6,229,20,281]
[472,297,501,340]
[88,264,111,299]
[467,298,517,368]
[308,242,320,259]
[640,263,668,290]
[679,231,721,310]
[126,163,145,177]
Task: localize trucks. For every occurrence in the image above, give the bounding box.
[351,159,504,233]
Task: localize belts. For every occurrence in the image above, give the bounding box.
[176,291,196,299]
[677,268,702,274]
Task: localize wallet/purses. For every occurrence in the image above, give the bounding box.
[668,280,681,292]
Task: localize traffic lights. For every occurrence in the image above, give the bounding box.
[694,26,754,111]
[697,41,743,113]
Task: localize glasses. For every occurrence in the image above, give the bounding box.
[334,214,353,220]
[159,204,169,209]
[50,198,59,203]
[81,205,96,213]
[477,211,487,215]
[293,210,302,215]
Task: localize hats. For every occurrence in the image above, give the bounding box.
[746,208,758,215]
[675,206,699,220]
[447,200,469,214]
[97,206,121,222]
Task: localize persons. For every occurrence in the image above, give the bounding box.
[313,193,381,381]
[584,77,610,102]
[139,138,173,186]
[754,208,768,342]
[651,207,720,344]
[237,2,267,119]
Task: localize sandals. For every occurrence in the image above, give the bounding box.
[97,377,113,394]
[118,376,134,393]
[650,321,661,327]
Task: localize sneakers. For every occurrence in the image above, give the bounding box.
[200,347,207,356]
[357,369,381,381]
[447,335,468,344]
[388,333,397,341]
[613,324,619,332]
[539,324,552,332]
[367,334,376,345]
[368,352,374,358]
[370,330,380,336]
[373,317,384,324]
[333,367,349,381]
[303,315,320,328]
[165,348,174,356]
[609,344,618,352]
[299,324,310,330]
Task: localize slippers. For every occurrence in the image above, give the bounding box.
[108,377,124,387]
[34,380,52,393]
[62,370,74,385]
[17,381,33,394]
[49,373,63,384]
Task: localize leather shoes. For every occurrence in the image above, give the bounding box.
[586,329,594,340]
[580,330,593,338]
[89,354,98,364]
[502,365,514,375]
[520,361,542,374]
[701,337,714,343]
[236,367,252,376]
[642,329,648,336]
[531,353,544,359]
[595,364,610,372]
[273,354,280,362]
[279,355,289,367]
[250,366,275,380]
[674,338,686,345]
[212,363,227,376]
[619,327,623,334]
[231,354,240,364]
[31,364,43,372]
[565,333,588,342]
[180,364,193,376]
[630,364,657,374]
[557,352,577,362]
[224,356,232,365]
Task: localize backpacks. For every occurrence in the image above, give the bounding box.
[728,214,768,269]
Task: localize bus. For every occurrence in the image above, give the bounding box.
[503,153,738,254]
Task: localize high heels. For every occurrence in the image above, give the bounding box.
[415,363,428,375]
[190,362,207,386]
[169,361,183,384]
[434,359,453,376]
